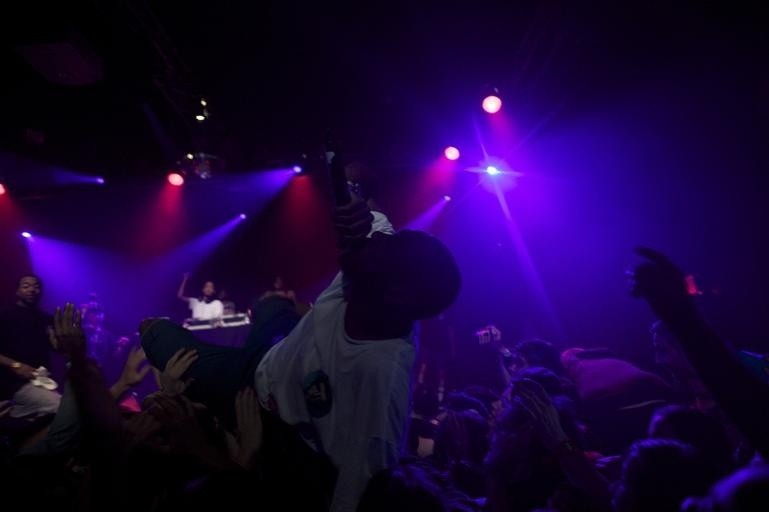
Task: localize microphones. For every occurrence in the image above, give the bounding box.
[331,154,356,249]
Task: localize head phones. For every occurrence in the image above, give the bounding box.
[198,295,214,303]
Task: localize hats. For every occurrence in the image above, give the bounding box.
[339,230,459,318]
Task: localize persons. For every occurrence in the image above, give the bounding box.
[241,187,460,510]
[1,241,767,512]
